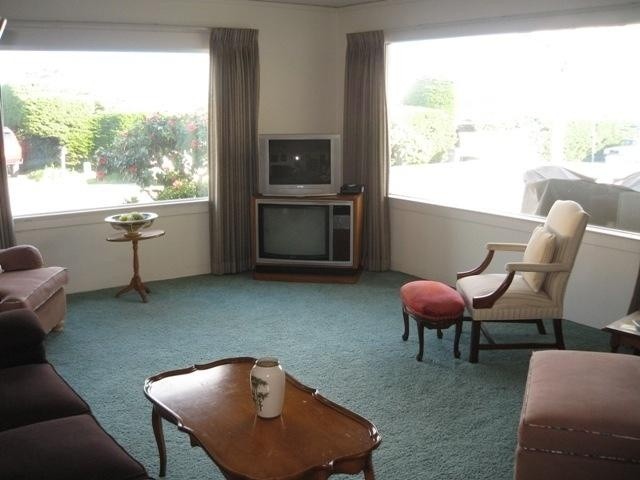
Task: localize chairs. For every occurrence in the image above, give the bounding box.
[453,197,591,362]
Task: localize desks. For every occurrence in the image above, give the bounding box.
[601,306,639,352]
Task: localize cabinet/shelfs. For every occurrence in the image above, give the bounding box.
[254,193,362,286]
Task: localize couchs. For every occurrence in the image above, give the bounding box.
[1,310,151,480]
[514,346,639,479]
[0,245,70,336]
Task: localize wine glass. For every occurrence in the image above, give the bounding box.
[103,211,159,237]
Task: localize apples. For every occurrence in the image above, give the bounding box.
[120,212,143,221]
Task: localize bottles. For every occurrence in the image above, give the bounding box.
[250,356,286,419]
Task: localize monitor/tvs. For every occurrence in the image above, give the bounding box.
[256,199,354,266]
[259,134,340,196]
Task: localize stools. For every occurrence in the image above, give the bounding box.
[402,280,464,363]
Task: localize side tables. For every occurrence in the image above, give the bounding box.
[106,228,165,303]
